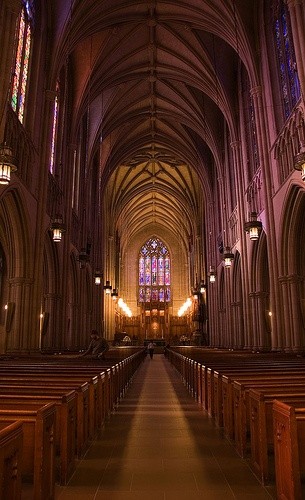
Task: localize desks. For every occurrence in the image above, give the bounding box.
[0,346,147,500]
[165,346,305,500]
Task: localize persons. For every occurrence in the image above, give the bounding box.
[148,341,156,359]
[122,335,131,346]
[80,330,109,359]
[180,335,188,345]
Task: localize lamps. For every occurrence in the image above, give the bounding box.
[193,211,263,299]
[47,214,118,300]
[0,141,20,185]
